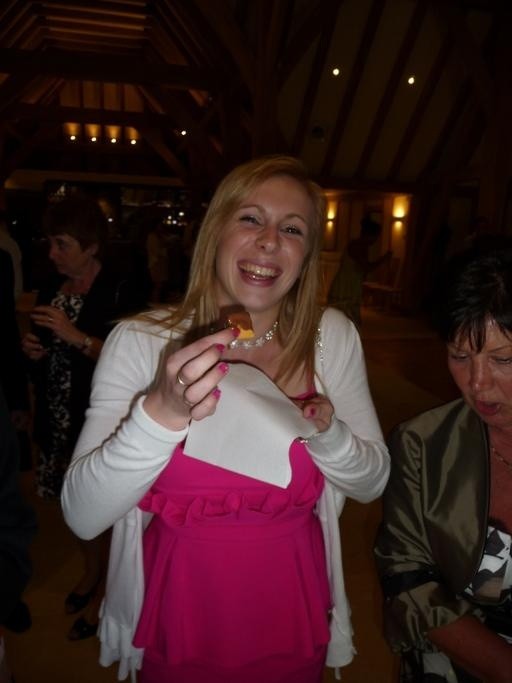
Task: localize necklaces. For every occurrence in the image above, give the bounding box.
[490,442,512,468]
[224,318,282,350]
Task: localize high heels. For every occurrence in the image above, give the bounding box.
[65,579,99,640]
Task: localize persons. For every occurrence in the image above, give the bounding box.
[1,200,119,641]
[60,156,391,683]
[371,273,512,681]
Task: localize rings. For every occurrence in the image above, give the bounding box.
[183,399,195,407]
[177,375,188,387]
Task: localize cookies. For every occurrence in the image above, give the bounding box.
[225,310,254,340]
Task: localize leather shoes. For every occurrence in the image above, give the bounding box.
[0,593,32,634]
